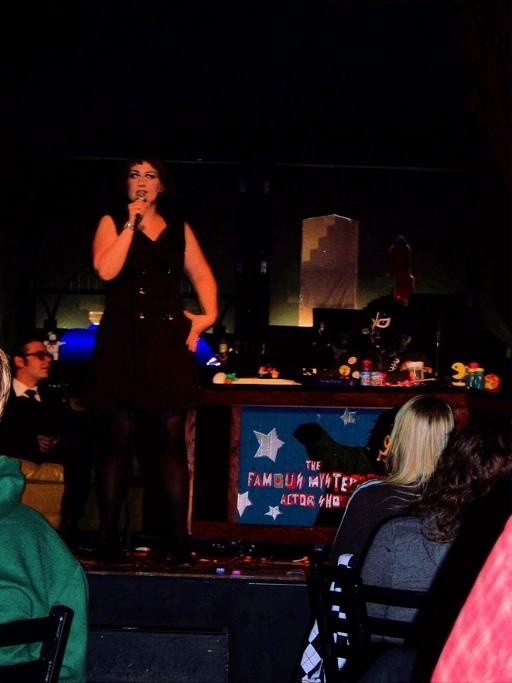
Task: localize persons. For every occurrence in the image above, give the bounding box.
[90,159,220,567]
[300,397,512,683]
[0,349,91,683]
[0,328,107,472]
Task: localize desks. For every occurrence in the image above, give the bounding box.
[185,384,502,547]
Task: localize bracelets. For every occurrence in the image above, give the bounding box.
[122,223,132,228]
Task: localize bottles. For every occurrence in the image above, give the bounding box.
[219,337,229,366]
[360,372,370,386]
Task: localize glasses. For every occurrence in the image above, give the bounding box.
[26,351,54,361]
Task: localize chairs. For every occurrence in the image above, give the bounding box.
[337,563,426,683]
[303,568,351,683]
[18,457,77,533]
[0,605,73,682]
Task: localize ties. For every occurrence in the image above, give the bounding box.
[25,390,36,399]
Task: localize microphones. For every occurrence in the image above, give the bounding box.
[134,192,146,226]
